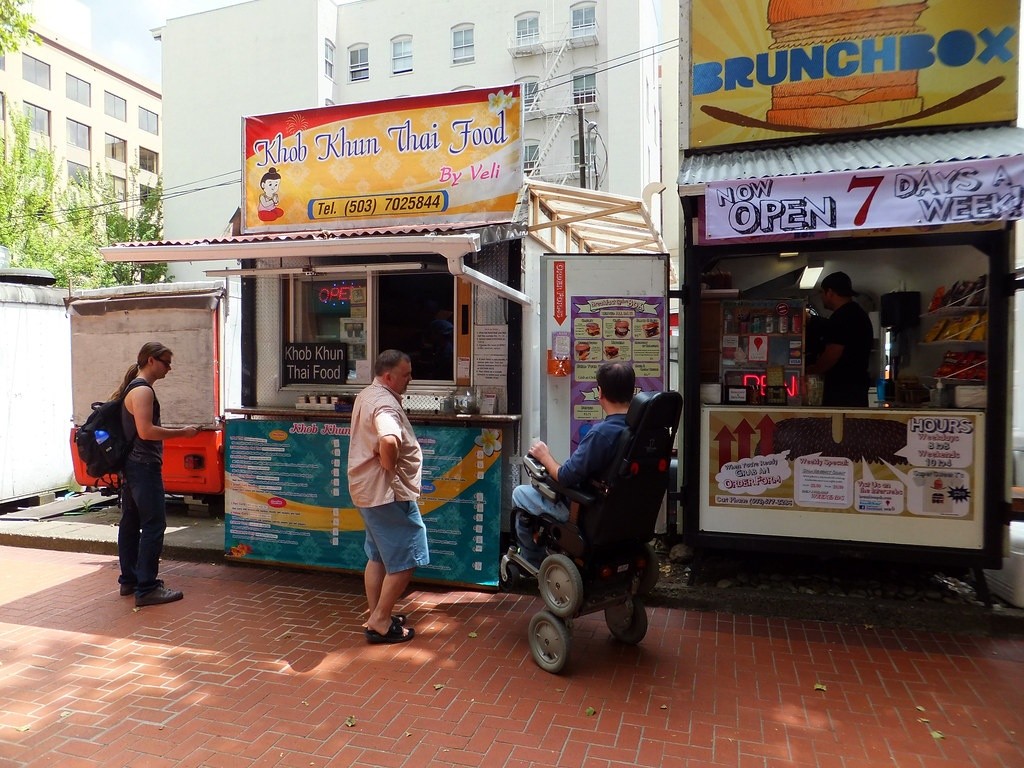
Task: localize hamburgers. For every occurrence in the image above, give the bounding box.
[586,323,599,336]
[615,321,629,337]
[575,344,590,360]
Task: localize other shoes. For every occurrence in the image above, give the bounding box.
[135,582,183,606]
[120,578,164,596]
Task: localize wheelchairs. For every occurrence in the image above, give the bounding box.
[498,388,684,674]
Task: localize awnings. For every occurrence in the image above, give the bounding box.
[99,221,528,262]
[678,126,1024,240]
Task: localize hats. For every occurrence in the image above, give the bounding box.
[821,271,860,297]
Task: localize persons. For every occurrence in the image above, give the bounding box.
[111,342,203,606]
[346,350,430,645]
[805,272,874,407]
[511,361,635,577]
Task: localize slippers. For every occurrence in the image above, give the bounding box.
[391,615,406,625]
[364,623,415,644]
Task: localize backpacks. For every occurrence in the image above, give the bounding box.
[74,382,160,478]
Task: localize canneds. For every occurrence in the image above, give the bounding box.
[765,315,774,333]
[752,315,761,333]
[778,316,788,333]
[738,321,747,333]
[791,315,802,333]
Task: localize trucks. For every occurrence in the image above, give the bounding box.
[68,279,240,518]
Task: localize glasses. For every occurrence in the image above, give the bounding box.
[154,356,171,367]
